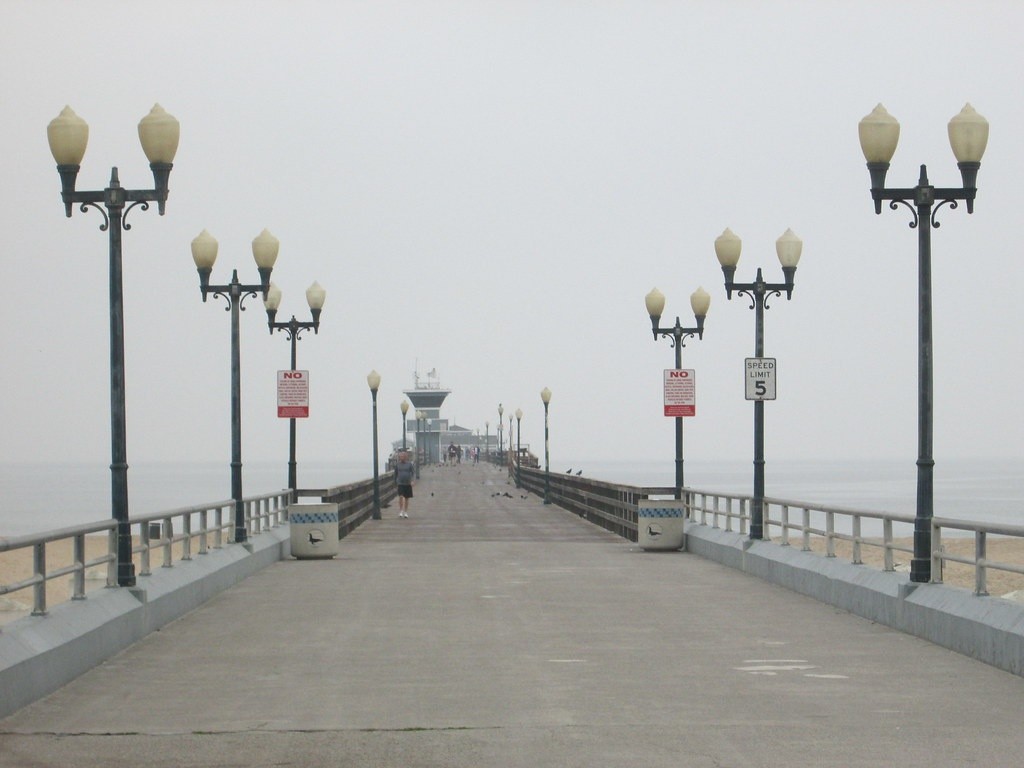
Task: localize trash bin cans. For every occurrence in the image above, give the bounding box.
[637,498,685,552]
[150,523,161,539]
[287,503,339,560]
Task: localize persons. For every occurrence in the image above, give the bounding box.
[441,441,480,467]
[393,451,417,518]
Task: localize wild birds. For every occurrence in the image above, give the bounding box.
[490,491,528,500]
[566,468,572,475]
[576,470,582,476]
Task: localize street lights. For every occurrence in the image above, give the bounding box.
[263,280,325,520]
[497,404,503,466]
[515,409,522,489]
[416,410,422,479]
[422,412,427,465]
[858,101,991,582]
[401,400,409,451]
[366,370,382,520]
[713,225,803,541]
[190,228,280,545]
[541,387,552,504]
[427,419,432,465]
[508,414,512,448]
[485,420,489,461]
[645,285,710,501]
[47,103,179,587]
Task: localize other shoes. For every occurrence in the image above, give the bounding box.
[404,512,409,517]
[399,511,404,517]
